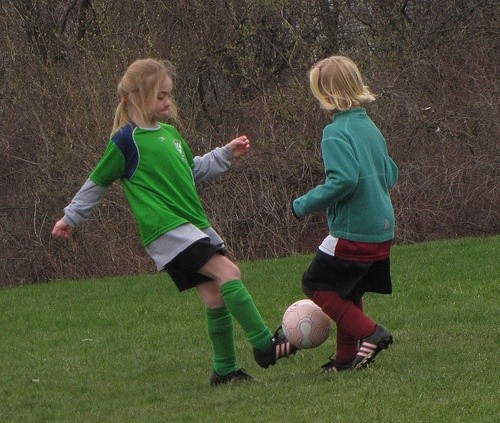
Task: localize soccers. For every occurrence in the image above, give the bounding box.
[281,298,330,350]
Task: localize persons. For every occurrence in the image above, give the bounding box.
[292,55,399,373]
[52,59,301,389]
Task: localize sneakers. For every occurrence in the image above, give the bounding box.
[210,368,262,386]
[253,325,301,369]
[319,353,356,375]
[351,322,394,372]
